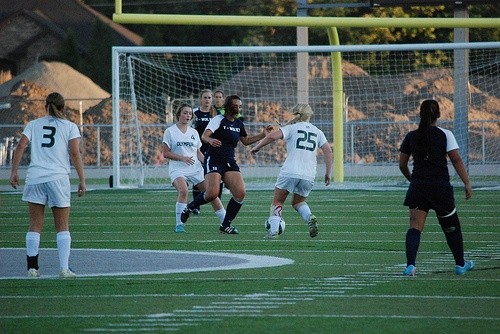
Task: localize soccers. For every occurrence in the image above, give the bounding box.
[264,216,286,235]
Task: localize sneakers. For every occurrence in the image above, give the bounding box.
[456,260,473,276]
[307,214,318,238]
[264,234,279,239]
[403,265,416,275]
[219,224,238,234]
[59,270,76,277]
[28,268,40,277]
[181,204,194,223]
[176,224,186,232]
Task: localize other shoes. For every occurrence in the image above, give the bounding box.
[193,209,200,214]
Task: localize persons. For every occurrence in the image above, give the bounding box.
[10,92,87,277]
[400,100,474,276]
[213,91,244,122]
[181,95,275,234]
[188,89,222,215]
[250,103,332,237]
[162,102,226,232]
